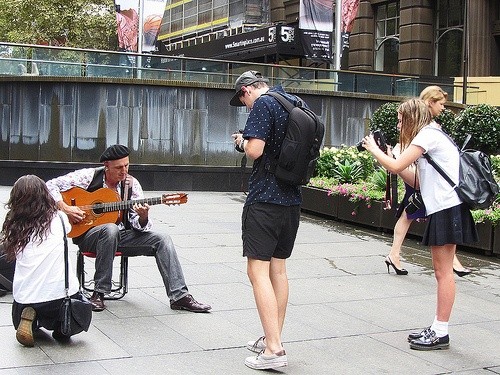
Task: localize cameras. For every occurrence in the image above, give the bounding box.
[234,129,245,154]
[356,129,388,153]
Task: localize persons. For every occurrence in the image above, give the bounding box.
[0,175,82,347]
[229,70,310,370]
[362,99,478,351]
[46,144,211,313]
[384,85,472,278]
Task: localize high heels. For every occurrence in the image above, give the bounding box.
[385,255,408,275]
[453,267,472,277]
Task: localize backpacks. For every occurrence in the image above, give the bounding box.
[260,91,324,184]
[422,127,498,210]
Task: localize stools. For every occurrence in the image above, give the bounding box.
[77,251,128,300]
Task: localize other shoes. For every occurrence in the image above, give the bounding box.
[16,307,36,346]
[52,327,69,342]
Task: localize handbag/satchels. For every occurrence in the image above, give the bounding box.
[405,195,424,215]
[58,297,93,336]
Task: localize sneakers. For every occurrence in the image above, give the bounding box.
[247,336,283,354]
[409,331,450,350]
[245,349,288,369]
[407,327,430,342]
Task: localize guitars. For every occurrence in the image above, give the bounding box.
[60,186,189,238]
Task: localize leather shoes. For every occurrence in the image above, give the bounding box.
[89,291,105,310]
[170,294,211,312]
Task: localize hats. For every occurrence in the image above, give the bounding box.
[99,145,131,163]
[229,70,268,106]
